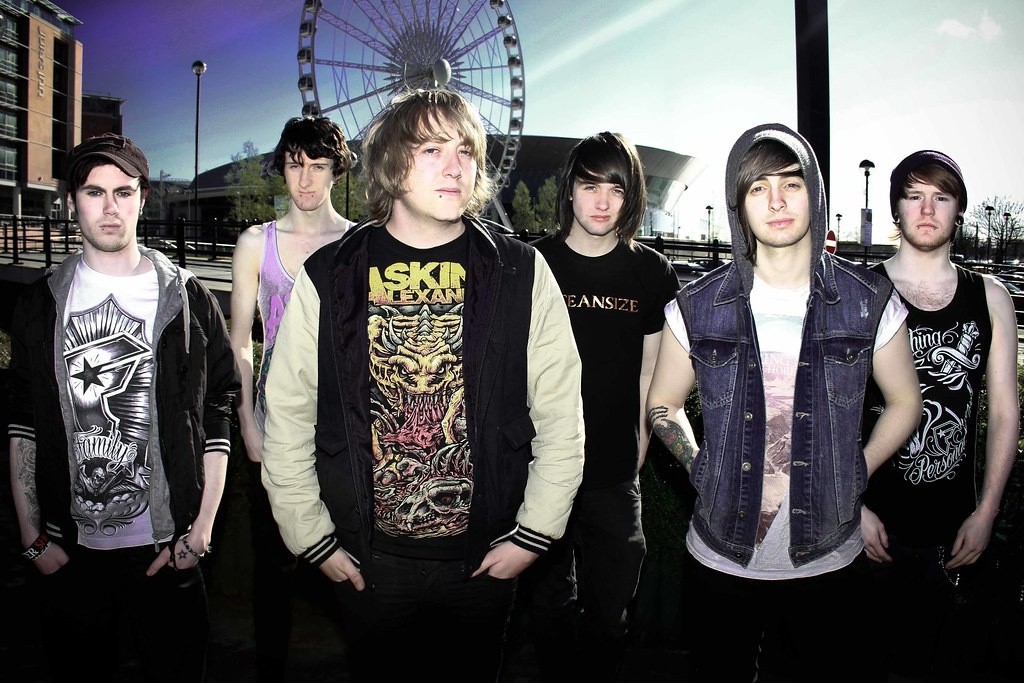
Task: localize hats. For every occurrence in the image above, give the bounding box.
[63,133,150,182]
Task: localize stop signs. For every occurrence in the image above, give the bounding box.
[825,230,837,254]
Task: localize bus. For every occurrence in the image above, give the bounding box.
[950,254,964,263]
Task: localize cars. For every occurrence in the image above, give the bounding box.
[963,256,993,269]
[689,258,725,272]
[668,261,708,281]
[1011,272,1024,280]
[1006,258,1020,265]
[991,276,1024,311]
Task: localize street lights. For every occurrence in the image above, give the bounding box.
[705,205,713,258]
[985,206,995,261]
[192,60,207,257]
[160,170,171,235]
[836,213,842,256]
[1003,212,1012,263]
[859,159,875,266]
[677,227,681,259]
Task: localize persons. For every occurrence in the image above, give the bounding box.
[860,151,1019,682]
[513,133,683,683]
[647,123,922,683]
[6,132,242,683]
[261,88,586,683]
[232,117,358,683]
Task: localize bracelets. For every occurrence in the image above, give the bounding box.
[22,533,51,560]
[182,536,206,559]
